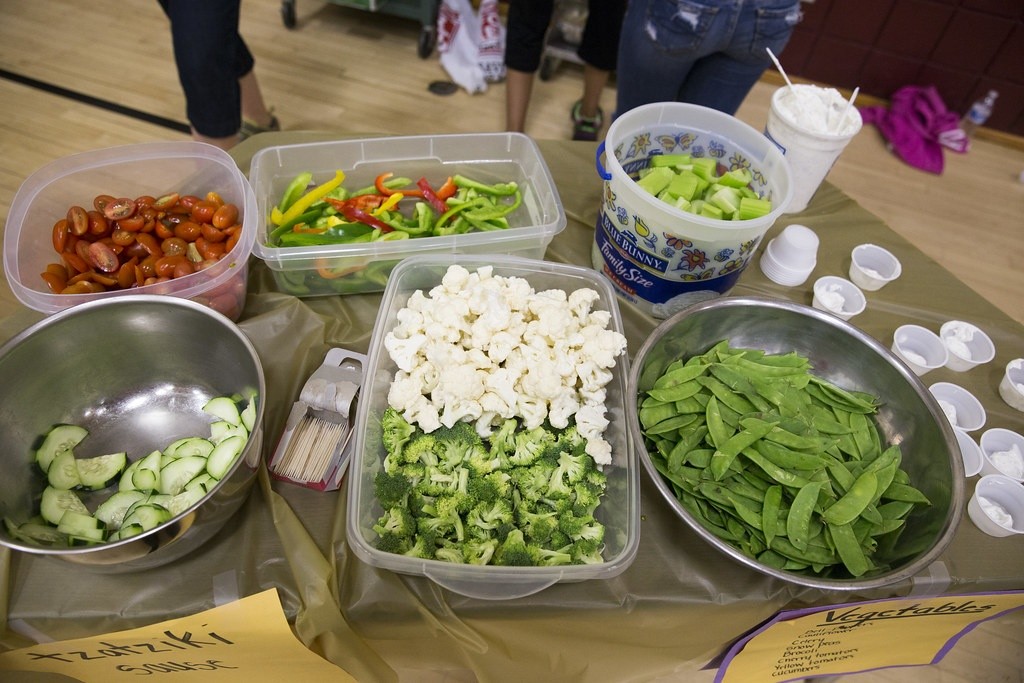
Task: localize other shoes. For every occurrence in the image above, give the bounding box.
[417,25,436,59]
[236,115,280,142]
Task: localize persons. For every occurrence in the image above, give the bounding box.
[617,0,802,115]
[156,1,279,155]
[503,0,625,142]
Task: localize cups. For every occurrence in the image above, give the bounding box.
[764,84,863,214]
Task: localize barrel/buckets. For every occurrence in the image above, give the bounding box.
[591,101,794,320]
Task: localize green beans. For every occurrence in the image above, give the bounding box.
[637,339,933,577]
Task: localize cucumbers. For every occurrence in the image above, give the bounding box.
[1,396,258,547]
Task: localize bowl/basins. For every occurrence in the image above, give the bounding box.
[952,428,984,477]
[0,294,266,574]
[979,428,1024,483]
[812,276,867,321]
[849,244,902,292]
[940,320,996,372]
[626,296,966,591]
[890,324,949,377]
[928,381,986,432]
[4,142,258,322]
[999,358,1024,412]
[968,474,1024,537]
[759,224,820,287]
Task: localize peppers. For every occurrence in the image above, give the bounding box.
[265,171,522,294]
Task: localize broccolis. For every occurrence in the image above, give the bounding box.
[372,263,628,566]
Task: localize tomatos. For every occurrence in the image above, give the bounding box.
[41,191,246,324]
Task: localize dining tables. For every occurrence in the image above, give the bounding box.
[0,131,1024,640]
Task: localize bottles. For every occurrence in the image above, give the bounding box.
[968,91,997,124]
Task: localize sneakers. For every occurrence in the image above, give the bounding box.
[572,101,604,141]
